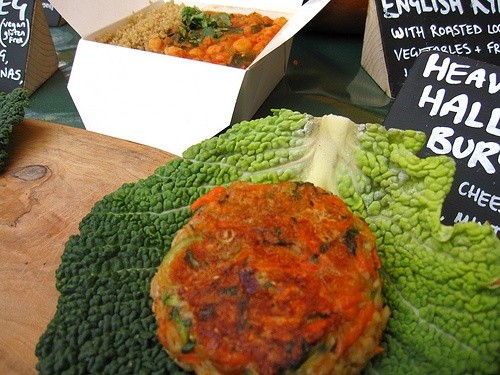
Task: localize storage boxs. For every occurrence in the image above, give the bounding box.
[47,0,333,159]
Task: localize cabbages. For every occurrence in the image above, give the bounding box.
[0,87,500,375]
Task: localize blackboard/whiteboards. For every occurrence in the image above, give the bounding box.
[0,0,59,102]
[39,0,68,28]
[380,50,499,240]
[360,0,500,100]
